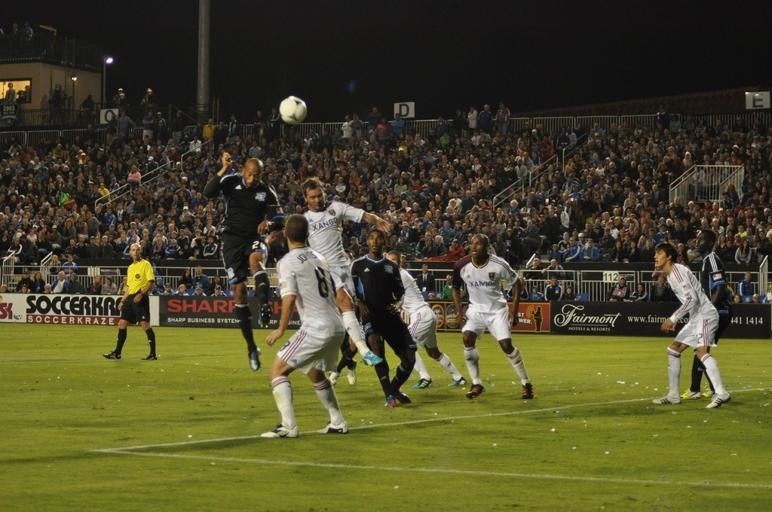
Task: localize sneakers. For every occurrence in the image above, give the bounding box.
[103,352,122,360]
[653,391,731,408]
[347,368,356,385]
[384,377,485,411]
[329,371,339,385]
[257,304,273,329]
[522,381,534,400]
[248,350,260,371]
[260,424,298,438]
[142,356,157,360]
[318,421,349,435]
[362,351,383,366]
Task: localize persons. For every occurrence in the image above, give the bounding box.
[648,241,734,411]
[349,229,417,406]
[677,228,734,401]
[296,177,392,366]
[203,151,281,373]
[388,250,467,390]
[259,213,349,437]
[450,233,536,400]
[327,248,360,386]
[1,74,771,304]
[102,242,159,360]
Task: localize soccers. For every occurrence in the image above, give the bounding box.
[280,95,307,123]
